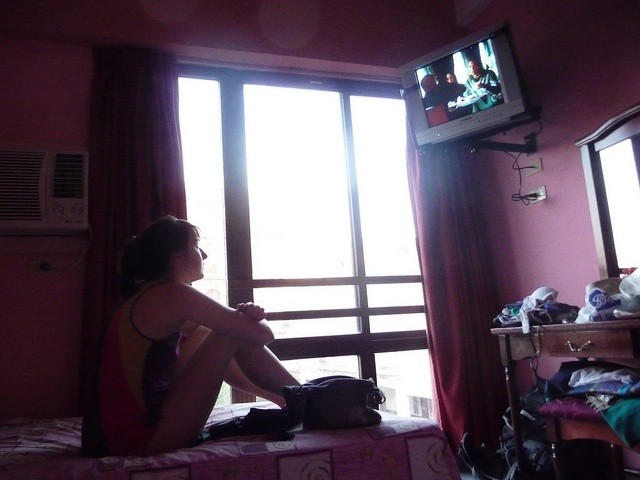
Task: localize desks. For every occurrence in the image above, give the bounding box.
[489,318,640,480]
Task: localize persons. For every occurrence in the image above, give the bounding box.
[420,73,453,114]
[465,59,502,99]
[91,213,303,458]
[444,72,465,102]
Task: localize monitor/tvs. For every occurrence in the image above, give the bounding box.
[398,21,527,147]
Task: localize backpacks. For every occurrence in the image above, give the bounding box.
[457,402,607,479]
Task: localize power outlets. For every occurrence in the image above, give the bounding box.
[525,158,543,175]
[528,186,549,204]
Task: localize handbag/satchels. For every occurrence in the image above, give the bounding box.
[281,376,385,430]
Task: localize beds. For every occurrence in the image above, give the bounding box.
[1,401,459,480]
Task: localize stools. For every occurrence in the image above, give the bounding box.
[537,397,640,480]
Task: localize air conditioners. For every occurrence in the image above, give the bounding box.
[0,149,89,237]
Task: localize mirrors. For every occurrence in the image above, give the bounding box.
[574,104,639,280]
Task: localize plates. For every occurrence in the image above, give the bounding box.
[462,95,473,101]
